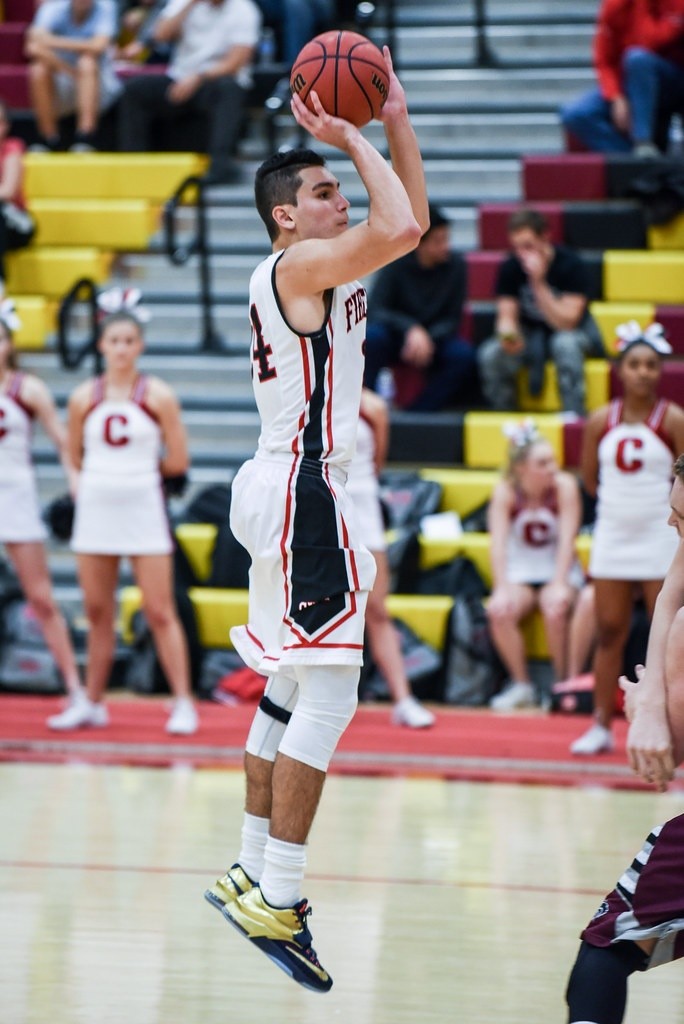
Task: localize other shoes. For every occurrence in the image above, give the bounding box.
[393,698,434,727]
[491,683,535,711]
[165,700,199,735]
[45,698,109,730]
[569,723,614,756]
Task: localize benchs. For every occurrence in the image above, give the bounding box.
[0,0,684,665]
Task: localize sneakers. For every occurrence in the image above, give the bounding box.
[204,864,333,995]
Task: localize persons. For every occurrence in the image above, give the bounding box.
[1,295,111,729]
[202,44,431,995]
[0,102,40,292]
[42,285,203,737]
[365,203,474,411]
[560,0,683,162]
[478,210,612,418]
[360,387,436,731]
[570,320,684,754]
[562,810,684,1024]
[615,454,684,792]
[24,0,261,185]
[484,418,580,711]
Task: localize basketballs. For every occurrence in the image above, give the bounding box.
[290,29,391,130]
